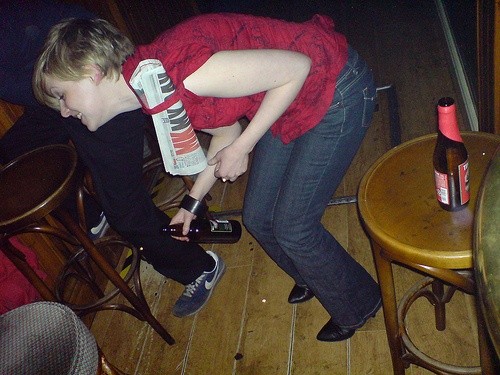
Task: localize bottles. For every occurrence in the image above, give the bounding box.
[159,219,243,244]
[433,96,470,212]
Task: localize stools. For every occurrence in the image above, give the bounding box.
[0,143,175,350]
[355,130,500,375]
[0,298,130,375]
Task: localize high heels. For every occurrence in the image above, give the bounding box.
[287,284,315,303]
[316,314,375,341]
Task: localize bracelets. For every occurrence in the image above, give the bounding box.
[178,194,209,218]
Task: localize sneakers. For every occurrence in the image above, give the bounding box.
[88,212,110,242]
[173,251,226,317]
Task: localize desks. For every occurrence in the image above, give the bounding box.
[471,143,500,362]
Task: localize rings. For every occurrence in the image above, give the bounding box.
[223,179,226,182]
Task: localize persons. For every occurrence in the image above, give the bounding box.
[0,0,226,315]
[32,13,382,343]
